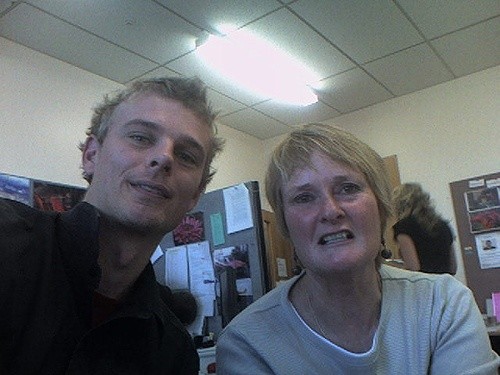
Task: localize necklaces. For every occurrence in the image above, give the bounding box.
[297,274,383,339]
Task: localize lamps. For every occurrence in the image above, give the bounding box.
[193,29,321,110]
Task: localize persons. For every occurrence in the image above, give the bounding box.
[215,122,500,375]
[1,74,228,375]
[390,181,459,276]
